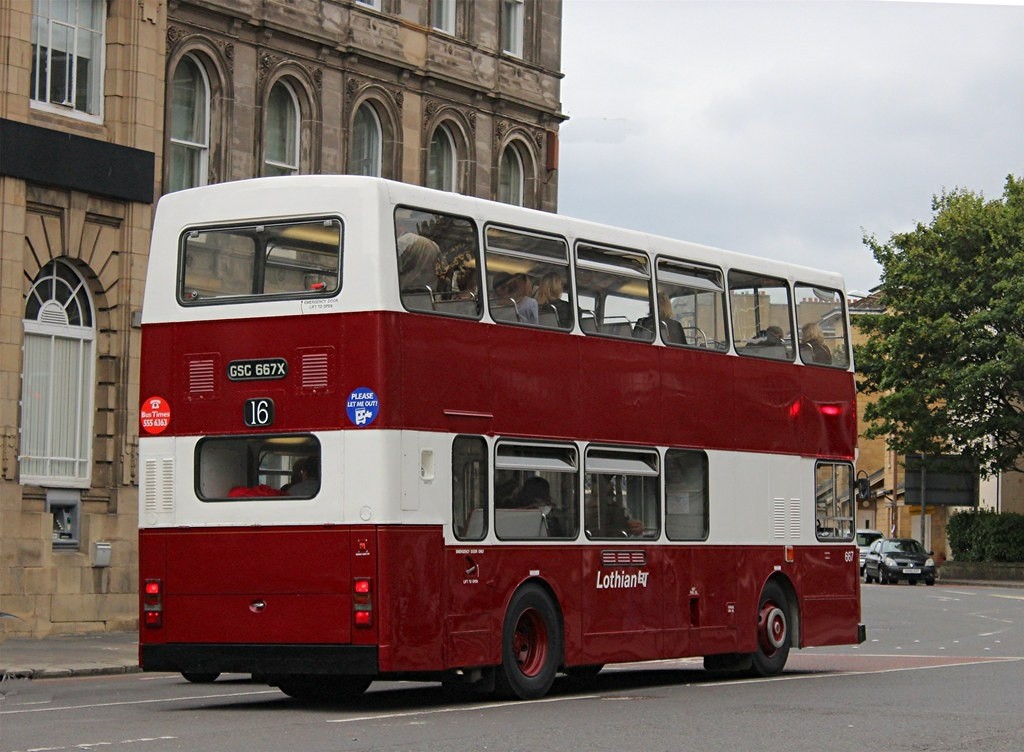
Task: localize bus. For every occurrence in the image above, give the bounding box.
[137,172,870,708]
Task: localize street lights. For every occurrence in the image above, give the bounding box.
[847,288,928,548]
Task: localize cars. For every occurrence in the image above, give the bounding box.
[862,537,936,585]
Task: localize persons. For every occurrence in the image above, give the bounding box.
[511,474,643,540]
[395,221,583,328]
[747,324,832,364]
[281,456,319,498]
[632,293,688,344]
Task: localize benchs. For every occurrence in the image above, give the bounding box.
[465,508,549,539]
[399,286,815,364]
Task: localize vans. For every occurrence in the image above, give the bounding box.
[838,529,886,571]
